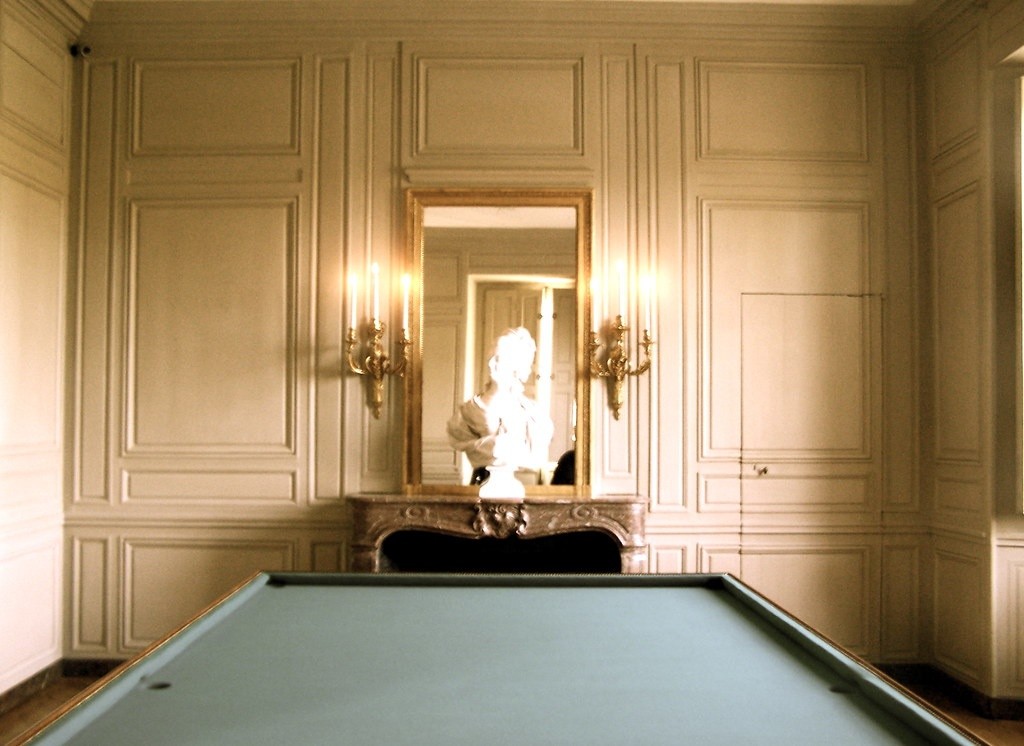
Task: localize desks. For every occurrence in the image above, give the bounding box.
[2,568,993,746]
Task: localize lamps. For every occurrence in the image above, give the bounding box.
[589,278,598,332]
[371,262,379,319]
[401,273,411,329]
[614,260,628,325]
[349,273,357,328]
[638,268,653,330]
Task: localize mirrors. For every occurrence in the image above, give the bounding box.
[404,185,594,496]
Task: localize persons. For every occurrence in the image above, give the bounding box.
[445,326,553,485]
